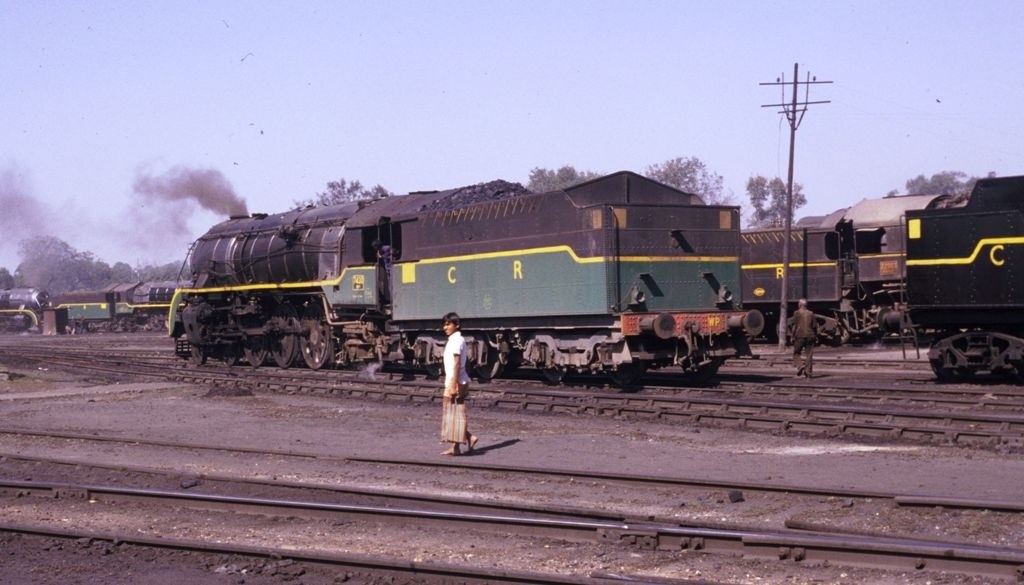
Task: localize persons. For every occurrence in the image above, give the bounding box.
[71,315,76,335]
[372,240,396,287]
[791,299,818,377]
[79,316,85,334]
[440,313,479,455]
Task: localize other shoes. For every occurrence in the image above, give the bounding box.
[796,364,805,377]
[805,373,812,379]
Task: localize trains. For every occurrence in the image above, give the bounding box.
[900,175,1024,385]
[0,195,966,351]
[168,170,767,391]
